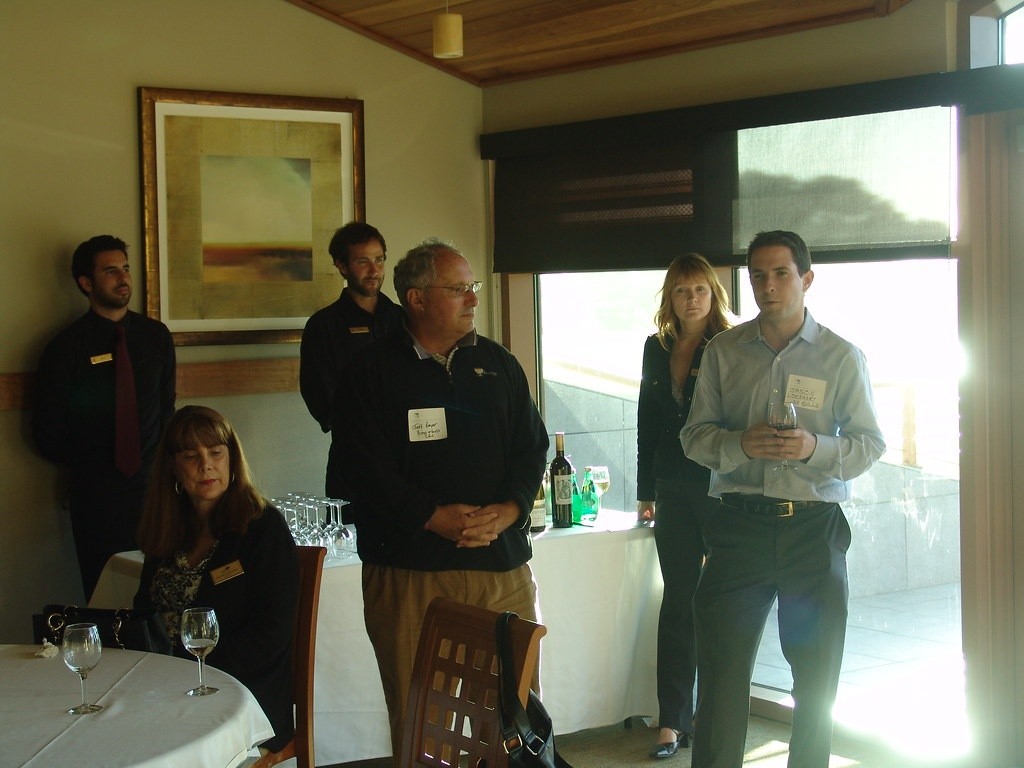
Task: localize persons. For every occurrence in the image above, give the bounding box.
[637,254,738,758]
[679,231,886,768]
[136,405,298,757]
[27,236,176,600]
[326,243,550,768]
[300,224,404,530]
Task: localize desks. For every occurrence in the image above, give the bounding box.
[88,507,654,729]
[0,644,276,768]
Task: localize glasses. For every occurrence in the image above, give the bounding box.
[429,281,483,296]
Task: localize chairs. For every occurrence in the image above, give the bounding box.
[315,595,546,768]
[237,547,327,768]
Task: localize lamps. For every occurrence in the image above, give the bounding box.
[431,0,464,58]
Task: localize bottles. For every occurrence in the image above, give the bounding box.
[542,462,552,515]
[529,483,546,532]
[571,470,582,523]
[581,468,598,521]
[550,433,573,528]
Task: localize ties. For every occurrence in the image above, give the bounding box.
[114,324,145,480]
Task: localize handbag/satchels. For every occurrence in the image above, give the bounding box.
[496,610,574,768]
[36,604,173,656]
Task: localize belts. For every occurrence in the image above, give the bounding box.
[719,492,825,518]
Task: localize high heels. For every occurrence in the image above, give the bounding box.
[648,729,691,758]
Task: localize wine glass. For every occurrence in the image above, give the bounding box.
[63,623,104,715]
[181,608,220,697]
[769,402,799,471]
[270,493,353,564]
[585,466,610,517]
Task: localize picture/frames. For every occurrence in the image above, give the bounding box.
[137,86,366,346]
